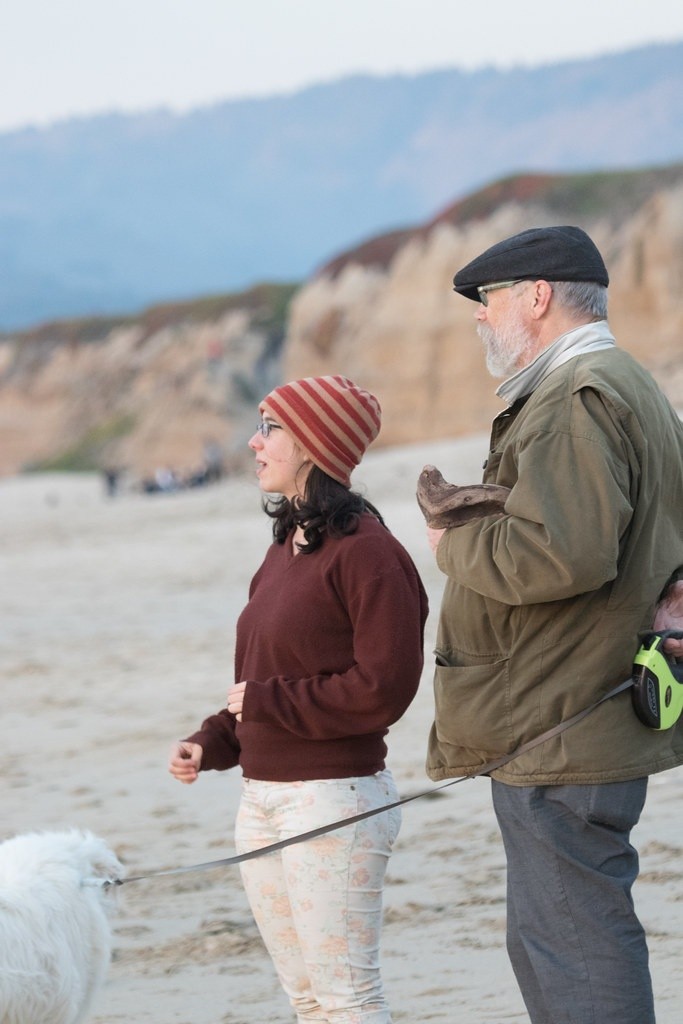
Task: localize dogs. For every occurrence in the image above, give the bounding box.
[0,826,125,1023]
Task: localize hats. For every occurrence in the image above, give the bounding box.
[259,375,382,488]
[453,225,609,303]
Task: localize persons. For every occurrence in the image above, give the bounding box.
[167,377,429,1024]
[424,226,683,1024]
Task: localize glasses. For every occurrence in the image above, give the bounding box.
[477,276,554,308]
[256,422,282,438]
